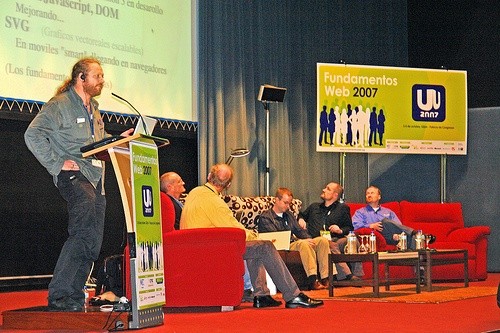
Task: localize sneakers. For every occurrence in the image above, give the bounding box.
[308,277,325,290]
[241,289,254,302]
[321,278,329,289]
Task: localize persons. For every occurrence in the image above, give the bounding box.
[159,172,255,303]
[179,163,324,309]
[352,186,436,286]
[258,187,339,291]
[297,182,365,288]
[24,57,134,311]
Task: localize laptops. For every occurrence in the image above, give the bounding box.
[132,115,157,136]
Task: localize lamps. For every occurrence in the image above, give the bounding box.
[226,147,251,166]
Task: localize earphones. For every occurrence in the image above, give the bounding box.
[81,75,85,79]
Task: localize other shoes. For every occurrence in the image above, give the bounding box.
[348,275,359,281]
[425,234,436,244]
[416,269,425,284]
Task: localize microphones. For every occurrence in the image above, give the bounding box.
[111,93,147,135]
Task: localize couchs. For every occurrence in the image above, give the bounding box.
[124,192,490,312]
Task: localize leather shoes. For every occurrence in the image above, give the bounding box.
[285,293,324,309]
[253,295,282,308]
[48,296,85,312]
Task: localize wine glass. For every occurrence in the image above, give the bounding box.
[358,235,367,253]
[344,235,348,254]
[365,235,370,253]
[424,234,431,249]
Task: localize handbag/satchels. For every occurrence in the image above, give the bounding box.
[94,254,123,299]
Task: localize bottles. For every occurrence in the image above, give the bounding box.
[400,230,407,251]
[370,231,376,254]
[416,230,424,250]
[347,231,356,254]
[82,286,89,312]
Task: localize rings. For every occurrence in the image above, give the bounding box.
[72,164,75,168]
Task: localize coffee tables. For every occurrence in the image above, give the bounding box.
[326,249,470,298]
